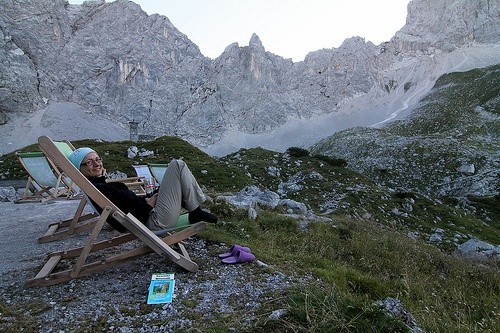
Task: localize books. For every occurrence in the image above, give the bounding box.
[146,273,174,304]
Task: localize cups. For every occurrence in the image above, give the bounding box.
[144,178,155,197]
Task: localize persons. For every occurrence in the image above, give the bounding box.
[68,147,218,234]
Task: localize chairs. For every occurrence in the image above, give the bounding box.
[14,135,207,286]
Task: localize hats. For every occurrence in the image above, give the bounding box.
[68,147,95,171]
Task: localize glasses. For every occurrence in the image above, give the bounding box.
[81,157,103,166]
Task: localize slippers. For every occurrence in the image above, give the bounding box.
[219,246,252,257]
[221,251,255,264]
